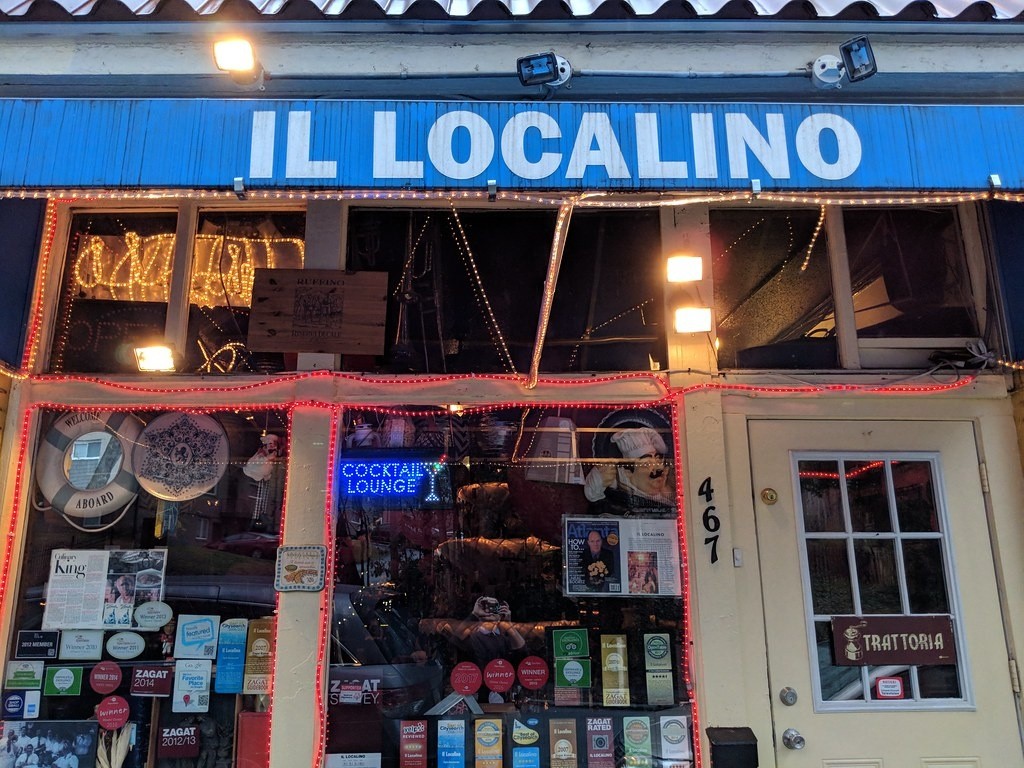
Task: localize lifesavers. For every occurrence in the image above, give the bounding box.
[36,411,144,518]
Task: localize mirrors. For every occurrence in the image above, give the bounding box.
[62,430,125,492]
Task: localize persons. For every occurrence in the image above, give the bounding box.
[160,617,176,655]
[577,530,614,592]
[116,576,134,604]
[0,726,92,768]
[447,592,531,703]
[242,434,279,482]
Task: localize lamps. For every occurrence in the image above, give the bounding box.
[344,408,382,448]
[812,34,878,90]
[213,37,264,92]
[525,407,587,485]
[517,53,572,91]
[134,343,190,373]
